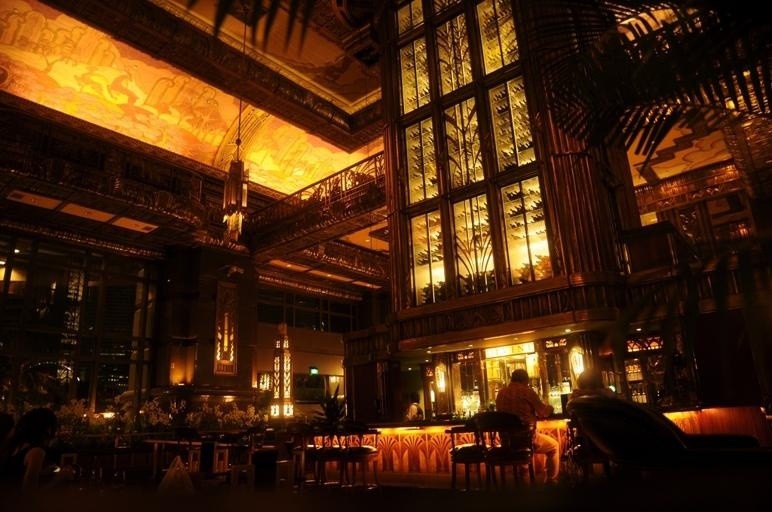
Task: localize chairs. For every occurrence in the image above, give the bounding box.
[287,427,381,493]
[565,395,758,485]
[220,427,255,487]
[445,408,536,491]
[167,427,204,456]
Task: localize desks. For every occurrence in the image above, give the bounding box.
[144,439,257,490]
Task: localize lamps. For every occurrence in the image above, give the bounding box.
[223,11,250,243]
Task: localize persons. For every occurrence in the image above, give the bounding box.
[403,391,424,420]
[495,368,559,489]
[576,366,604,392]
[0,408,58,512]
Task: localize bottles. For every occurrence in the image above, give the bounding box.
[602,364,647,404]
